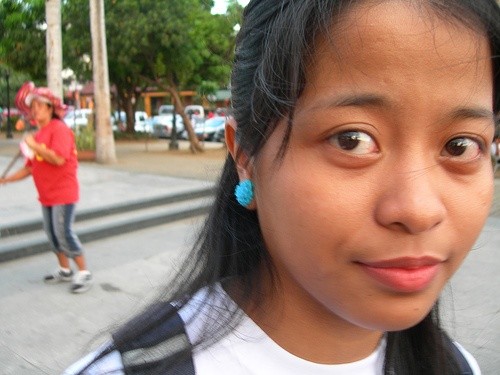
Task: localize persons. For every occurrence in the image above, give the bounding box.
[61,0,500,374]
[0,82,93,292]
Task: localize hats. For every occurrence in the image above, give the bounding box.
[15,83,68,122]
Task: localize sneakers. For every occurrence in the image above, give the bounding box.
[70,268,94,293]
[44,267,73,283]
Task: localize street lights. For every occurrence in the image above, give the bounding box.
[3,65,13,139]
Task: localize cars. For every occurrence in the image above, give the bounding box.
[63,105,227,143]
